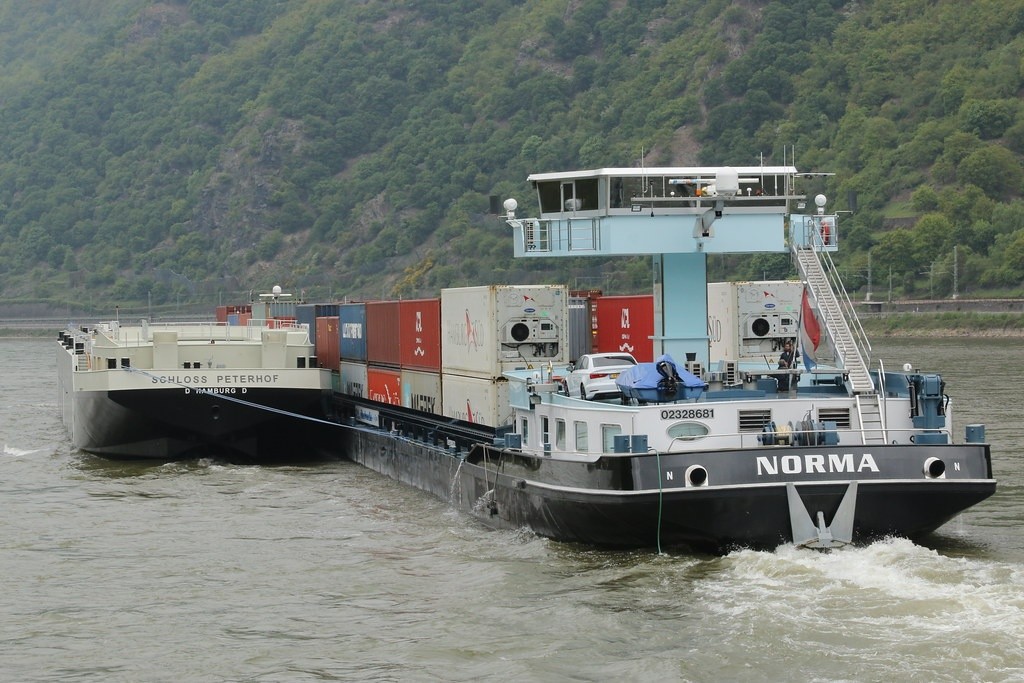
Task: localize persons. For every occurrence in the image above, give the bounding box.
[766,358,797,391]
[820,221,830,245]
[779,342,800,369]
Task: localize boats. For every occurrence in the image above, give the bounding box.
[215,166,999,557]
[614,354,704,400]
[57,318,333,457]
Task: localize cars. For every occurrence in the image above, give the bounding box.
[563,353,637,401]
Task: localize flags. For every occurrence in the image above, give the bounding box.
[800,285,820,374]
